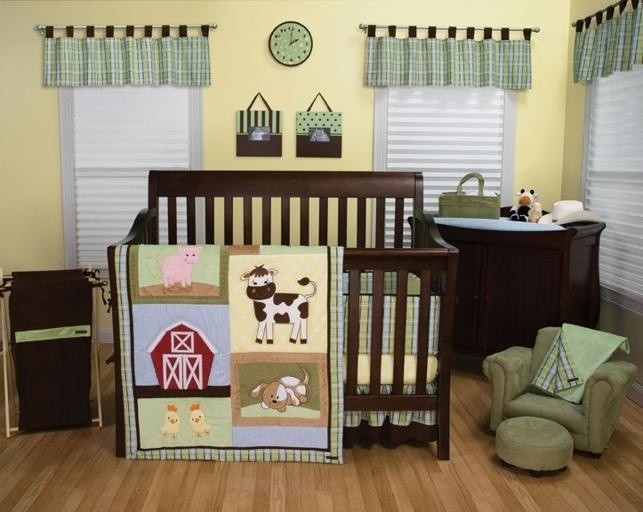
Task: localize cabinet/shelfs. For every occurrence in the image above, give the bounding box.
[407,204,607,371]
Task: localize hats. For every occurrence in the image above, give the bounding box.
[538,200,600,225]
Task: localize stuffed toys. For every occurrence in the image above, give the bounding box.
[508,187,535,222]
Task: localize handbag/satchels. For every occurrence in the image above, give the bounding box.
[235,93,283,157]
[296,93,343,158]
[439,173,500,219]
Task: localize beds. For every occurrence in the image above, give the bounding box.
[104,162,463,468]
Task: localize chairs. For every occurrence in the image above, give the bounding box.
[481,319,638,461]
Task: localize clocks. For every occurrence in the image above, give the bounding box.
[269,19,313,69]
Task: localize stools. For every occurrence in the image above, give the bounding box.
[493,416,576,479]
